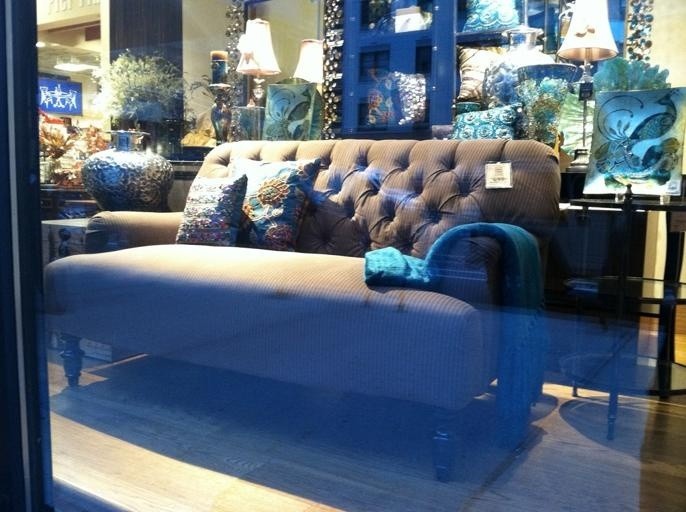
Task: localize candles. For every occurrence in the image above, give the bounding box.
[211,51,229,61]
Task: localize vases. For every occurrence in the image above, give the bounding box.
[81,131,174,210]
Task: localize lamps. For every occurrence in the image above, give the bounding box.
[293,38,325,89]
[557,0,619,166]
[235,18,281,140]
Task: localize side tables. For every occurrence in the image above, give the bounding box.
[569,198,686,441]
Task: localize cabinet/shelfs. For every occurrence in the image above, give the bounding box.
[42,218,145,365]
[324,0,511,140]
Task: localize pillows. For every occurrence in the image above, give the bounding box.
[362,67,401,130]
[457,45,506,102]
[176,174,248,247]
[229,155,320,253]
[448,102,524,141]
[461,0,519,33]
[393,72,426,127]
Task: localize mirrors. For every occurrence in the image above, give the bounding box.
[226,0,343,139]
[516,0,654,152]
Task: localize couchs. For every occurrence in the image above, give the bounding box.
[43,139,562,485]
